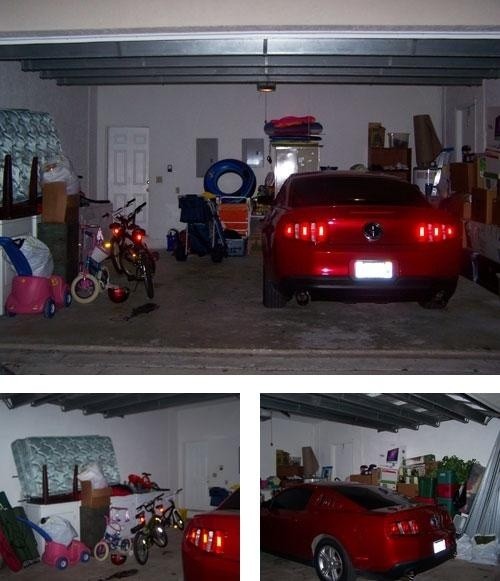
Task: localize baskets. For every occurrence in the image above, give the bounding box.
[91,245,110,263]
[107,522,121,536]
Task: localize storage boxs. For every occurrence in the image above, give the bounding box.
[75,480,113,511]
[345,443,469,536]
[445,146,500,295]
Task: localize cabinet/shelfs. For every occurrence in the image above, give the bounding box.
[276,463,307,495]
[17,488,175,563]
[367,146,412,186]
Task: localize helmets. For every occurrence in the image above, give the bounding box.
[107,286,131,303]
[110,551,128,566]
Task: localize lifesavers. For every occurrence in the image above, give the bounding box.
[204,158,256,204]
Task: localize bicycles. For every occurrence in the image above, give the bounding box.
[71,219,112,307]
[102,197,137,275]
[93,513,132,564]
[109,201,157,300]
[130,493,169,568]
[150,488,185,535]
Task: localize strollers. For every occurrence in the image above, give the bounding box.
[173,193,226,263]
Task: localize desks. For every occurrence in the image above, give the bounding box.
[22,462,85,506]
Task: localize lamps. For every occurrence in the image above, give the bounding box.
[256,81,277,93]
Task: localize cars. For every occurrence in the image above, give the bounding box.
[181,485,240,581]
[260,480,457,580]
[253,169,473,314]
